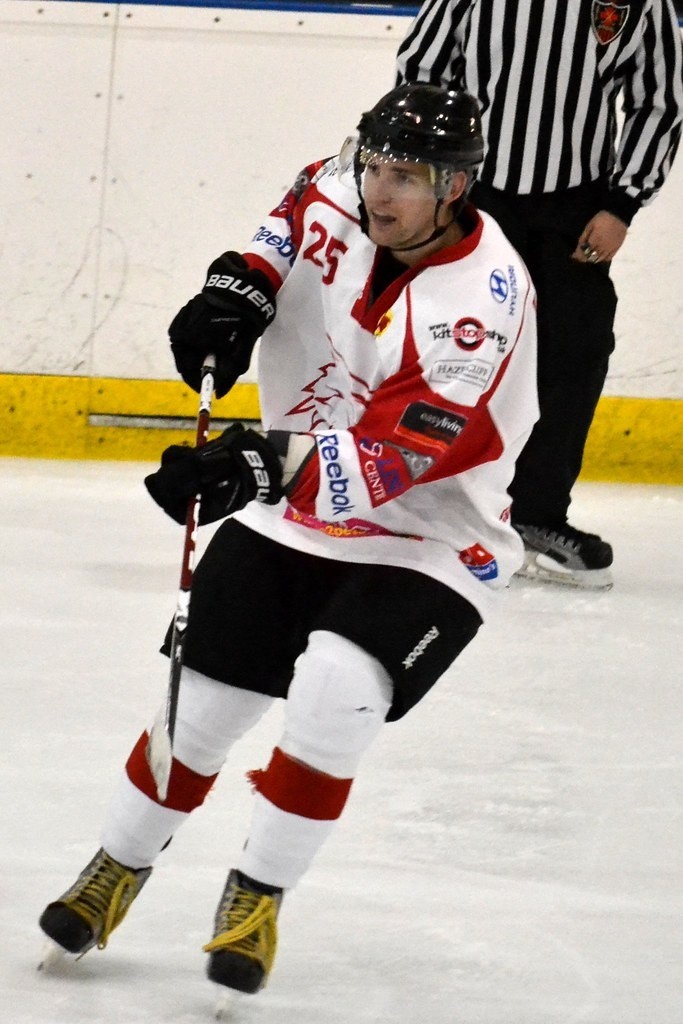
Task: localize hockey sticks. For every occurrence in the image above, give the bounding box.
[142,357,217,802]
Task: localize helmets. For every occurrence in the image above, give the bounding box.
[357,80,485,202]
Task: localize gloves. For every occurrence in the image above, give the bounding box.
[168,251,277,399]
[144,422,283,527]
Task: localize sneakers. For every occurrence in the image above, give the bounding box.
[509,518,617,591]
[36,844,152,972]
[204,867,286,1022]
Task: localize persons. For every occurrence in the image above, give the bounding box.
[392,0,683,585]
[39,83,544,992]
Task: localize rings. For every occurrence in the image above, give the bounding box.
[583,247,591,257]
[589,250,599,262]
